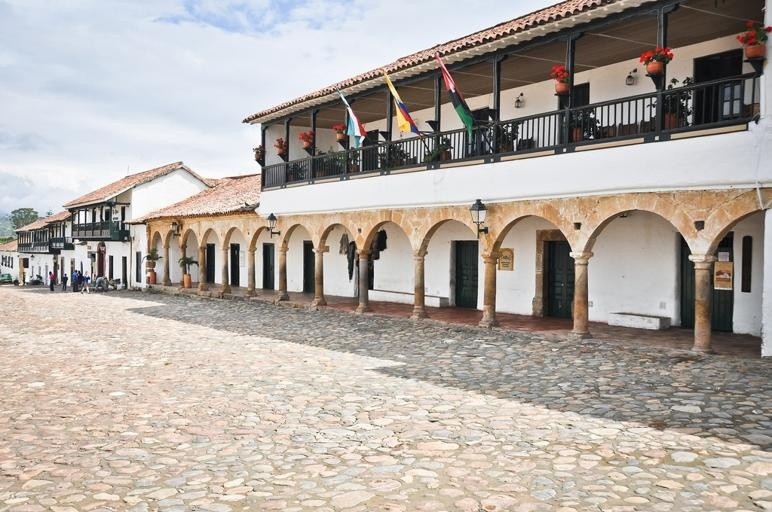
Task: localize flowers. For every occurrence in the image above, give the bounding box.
[736,20,772,47]
[548,65,569,83]
[640,47,673,65]
[275,137,287,148]
[298,130,314,141]
[333,123,346,134]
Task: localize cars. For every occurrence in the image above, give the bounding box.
[0,273,12,284]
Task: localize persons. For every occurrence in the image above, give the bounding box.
[48,271,56,292]
[71,270,91,294]
[62,273,69,292]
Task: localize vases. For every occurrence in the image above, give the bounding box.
[647,62,663,74]
[278,149,283,152]
[744,44,766,58]
[304,141,311,147]
[555,83,569,93]
[337,133,346,140]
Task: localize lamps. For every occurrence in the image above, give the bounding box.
[626,69,637,85]
[470,199,488,239]
[170,222,181,239]
[266,213,281,238]
[515,93,523,108]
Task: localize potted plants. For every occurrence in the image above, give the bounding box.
[497,122,521,152]
[178,256,198,288]
[438,137,454,161]
[146,248,163,283]
[647,77,695,128]
[571,109,600,141]
[287,145,407,182]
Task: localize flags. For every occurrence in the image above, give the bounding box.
[434,53,476,135]
[335,86,368,151]
[383,70,422,139]
